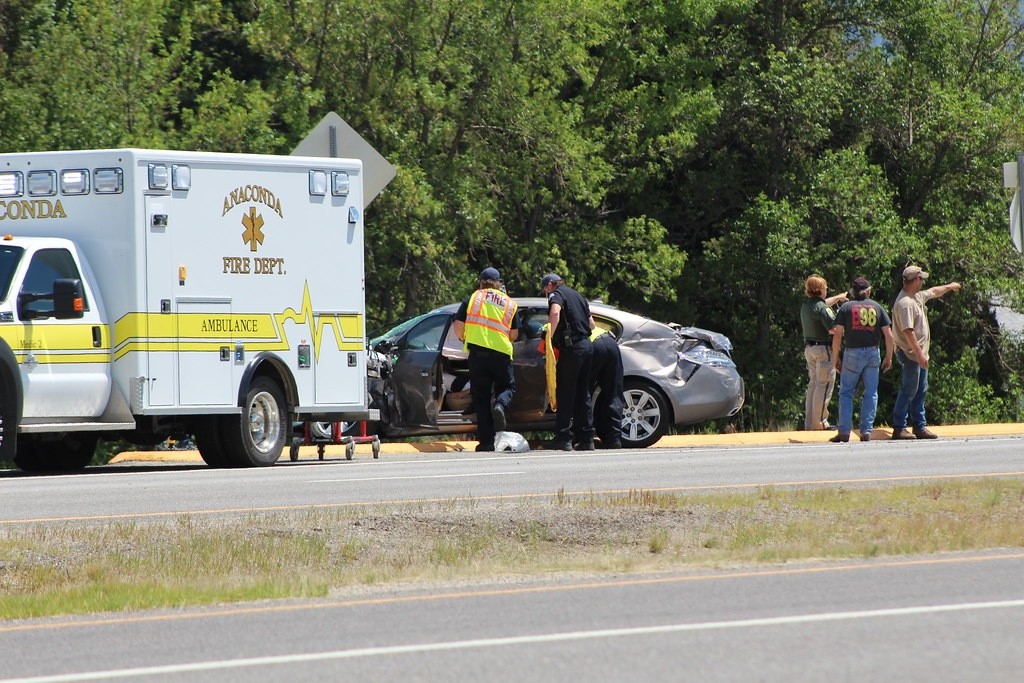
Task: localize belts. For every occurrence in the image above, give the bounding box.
[808,341,832,345]
[596,334,610,338]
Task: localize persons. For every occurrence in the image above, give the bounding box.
[451,267,523,452]
[801,275,850,431]
[892,265,961,439]
[829,278,894,443]
[536,273,596,451]
[589,325,624,449]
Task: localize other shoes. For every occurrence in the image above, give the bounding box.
[829,434,849,442]
[597,441,622,449]
[493,403,508,432]
[541,437,573,451]
[475,443,495,451]
[860,434,870,442]
[574,442,595,451]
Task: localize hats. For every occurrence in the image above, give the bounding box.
[852,277,871,291]
[542,274,562,289]
[480,267,500,281]
[902,265,929,280]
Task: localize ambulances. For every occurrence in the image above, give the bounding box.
[1,148,380,473]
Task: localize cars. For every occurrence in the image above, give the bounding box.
[312,296,743,445]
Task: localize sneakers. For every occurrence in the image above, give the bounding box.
[891,428,917,440]
[912,429,938,440]
